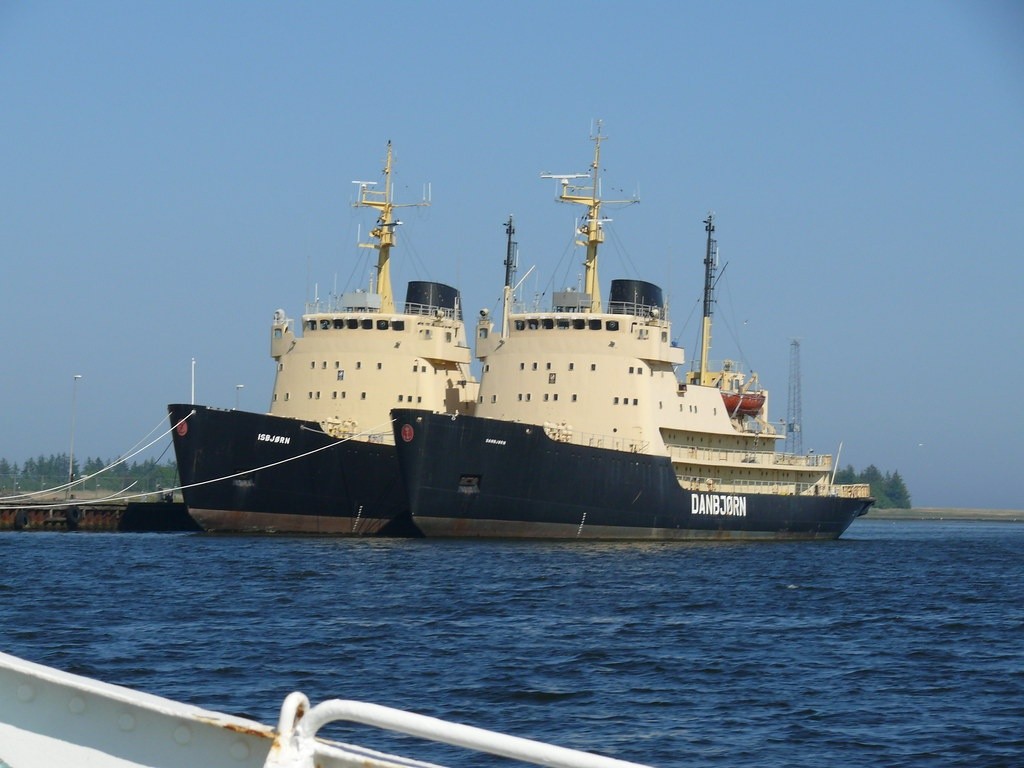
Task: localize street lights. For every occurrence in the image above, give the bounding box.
[65,375,83,499]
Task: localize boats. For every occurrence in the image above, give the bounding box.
[387,119,877,542]
[166,138,478,534]
